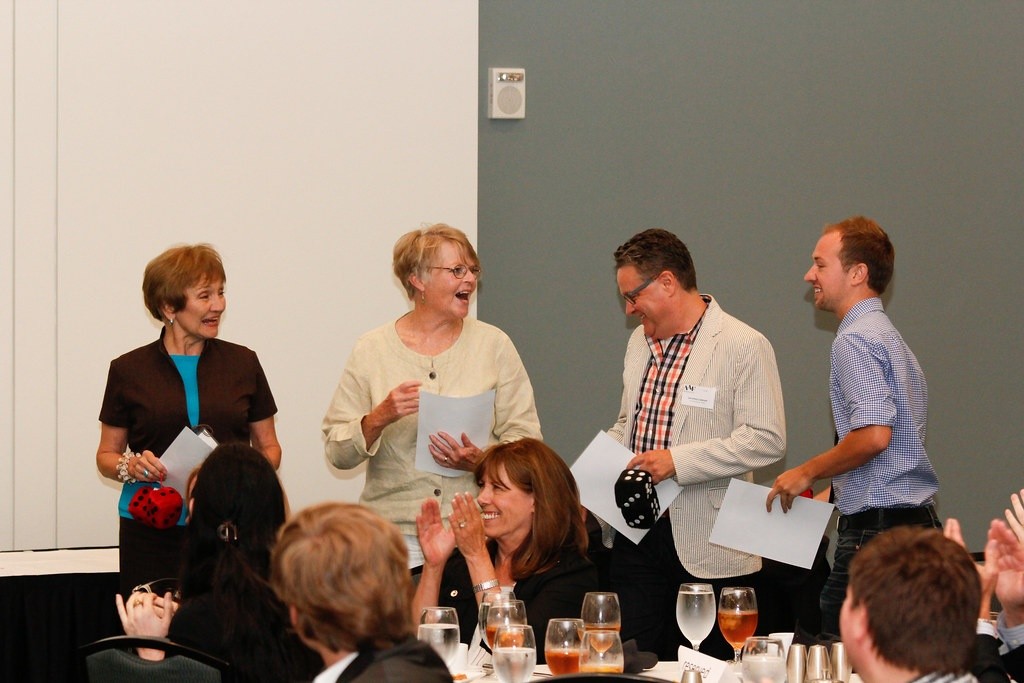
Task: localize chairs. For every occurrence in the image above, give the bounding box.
[75,635,229,683]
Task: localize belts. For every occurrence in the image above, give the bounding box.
[837,505,936,532]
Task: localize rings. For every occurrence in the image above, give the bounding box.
[143,470,149,477]
[444,456,448,463]
[133,599,143,607]
[459,521,467,528]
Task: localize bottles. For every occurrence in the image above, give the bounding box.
[742,636,787,683]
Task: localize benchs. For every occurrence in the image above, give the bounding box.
[0,572,119,683]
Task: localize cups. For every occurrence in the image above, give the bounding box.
[420,606,460,633]
[477,591,528,660]
[491,625,538,683]
[786,640,854,683]
[681,669,702,683]
[578,630,624,676]
[545,618,586,675]
[417,622,460,676]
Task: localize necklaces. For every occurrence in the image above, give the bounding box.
[411,311,455,394]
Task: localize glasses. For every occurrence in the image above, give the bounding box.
[428,264,480,278]
[623,280,651,304]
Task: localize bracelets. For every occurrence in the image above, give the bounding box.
[116,452,141,484]
[472,578,499,594]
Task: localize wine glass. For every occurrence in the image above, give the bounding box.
[676,582,717,654]
[718,587,758,666]
[580,590,622,664]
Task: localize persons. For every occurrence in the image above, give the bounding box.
[268,504,453,683]
[582,228,787,661]
[767,215,944,652]
[411,438,599,664]
[321,222,544,575]
[839,526,980,683]
[96,243,282,604]
[945,490,1024,683]
[115,442,326,683]
